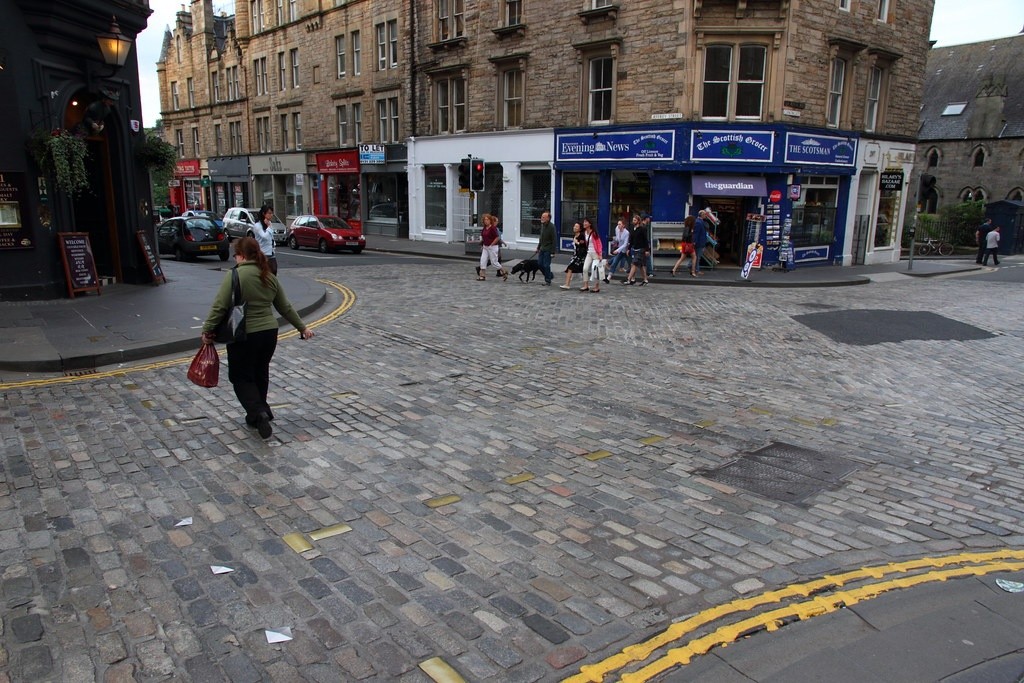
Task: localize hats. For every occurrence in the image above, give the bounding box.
[641,213,653,219]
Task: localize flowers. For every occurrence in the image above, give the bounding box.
[29,126,94,200]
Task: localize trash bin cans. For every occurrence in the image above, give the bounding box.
[464,228,484,255]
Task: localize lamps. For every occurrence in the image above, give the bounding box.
[592,125,851,143]
[91,14,133,82]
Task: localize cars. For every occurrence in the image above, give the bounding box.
[288,214,366,254]
[425,203,447,226]
[181,210,223,230]
[222,207,289,246]
[369,203,408,220]
[157,216,229,261]
[185,194,243,207]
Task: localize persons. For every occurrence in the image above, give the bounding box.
[536,211,557,286]
[253,204,278,276]
[669,210,707,277]
[201,237,316,442]
[975,218,1001,266]
[160,201,180,218]
[476,213,509,281]
[579,215,603,293]
[560,221,587,290]
[602,213,657,287]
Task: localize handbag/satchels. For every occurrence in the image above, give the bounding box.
[213,267,247,343]
[187,332,220,388]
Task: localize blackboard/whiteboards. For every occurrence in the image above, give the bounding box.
[138,230,164,280]
[58,232,100,292]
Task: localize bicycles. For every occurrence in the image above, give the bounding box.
[918,236,954,257]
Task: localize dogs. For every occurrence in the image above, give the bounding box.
[511,259,554,282]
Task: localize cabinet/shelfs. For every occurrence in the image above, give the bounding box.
[650,221,693,268]
[702,217,718,269]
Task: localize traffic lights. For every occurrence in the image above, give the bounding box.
[458,159,470,189]
[470,158,484,191]
[919,173,936,201]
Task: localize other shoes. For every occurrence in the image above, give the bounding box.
[603,279,609,284]
[620,279,636,285]
[560,284,570,290]
[639,281,648,287]
[688,269,704,277]
[496,272,503,277]
[245,408,274,439]
[606,261,610,271]
[476,276,485,281]
[579,287,589,291]
[618,268,627,273]
[669,270,676,278]
[541,282,551,286]
[590,289,600,293]
[502,271,508,281]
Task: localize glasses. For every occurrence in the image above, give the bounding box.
[233,253,240,260]
